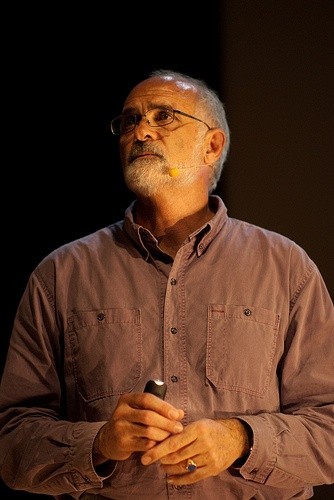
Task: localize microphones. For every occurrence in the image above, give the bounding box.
[169,164,211,178]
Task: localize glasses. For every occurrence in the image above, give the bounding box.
[110,105,210,135]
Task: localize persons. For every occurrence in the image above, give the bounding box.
[0,68,334,500]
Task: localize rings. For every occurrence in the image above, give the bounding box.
[186,459,197,472]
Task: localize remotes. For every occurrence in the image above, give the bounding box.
[143,380,167,401]
